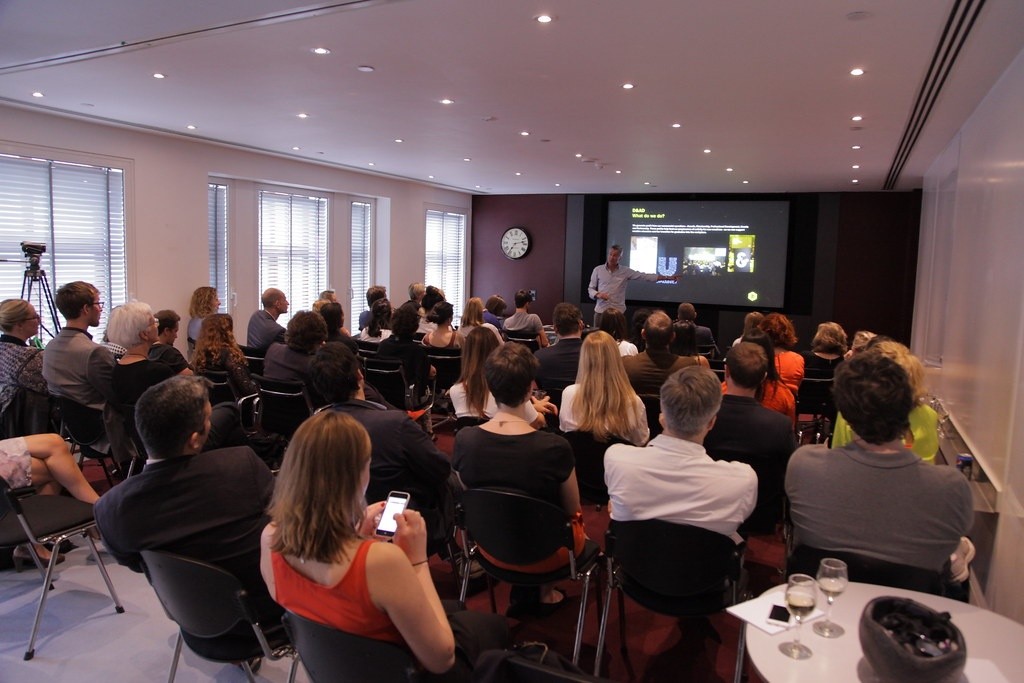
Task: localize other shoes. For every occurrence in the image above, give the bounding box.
[539,587,568,615]
[459,559,485,580]
[445,556,462,573]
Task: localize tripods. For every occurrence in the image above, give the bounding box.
[0,253,61,342]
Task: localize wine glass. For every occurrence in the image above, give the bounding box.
[814,557,848,637]
[783,573,819,657]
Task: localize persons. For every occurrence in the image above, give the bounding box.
[0,281,975,683]
[682,257,726,277]
[588,244,682,329]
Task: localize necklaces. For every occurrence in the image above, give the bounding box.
[129,353,148,360]
[490,418,528,428]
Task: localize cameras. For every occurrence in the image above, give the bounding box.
[21,241,46,253]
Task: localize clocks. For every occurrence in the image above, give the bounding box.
[499,227,532,261]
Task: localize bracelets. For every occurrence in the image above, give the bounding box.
[412,559,428,566]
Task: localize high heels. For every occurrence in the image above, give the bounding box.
[12,544,65,572]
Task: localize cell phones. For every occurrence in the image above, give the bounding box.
[374,490,410,539]
[766,601,791,627]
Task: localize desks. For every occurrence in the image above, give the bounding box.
[746,579,1024,683]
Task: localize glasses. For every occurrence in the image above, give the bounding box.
[147,318,159,327]
[81,302,104,310]
[14,314,40,325]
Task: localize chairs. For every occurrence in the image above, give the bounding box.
[0,331,952,683]
